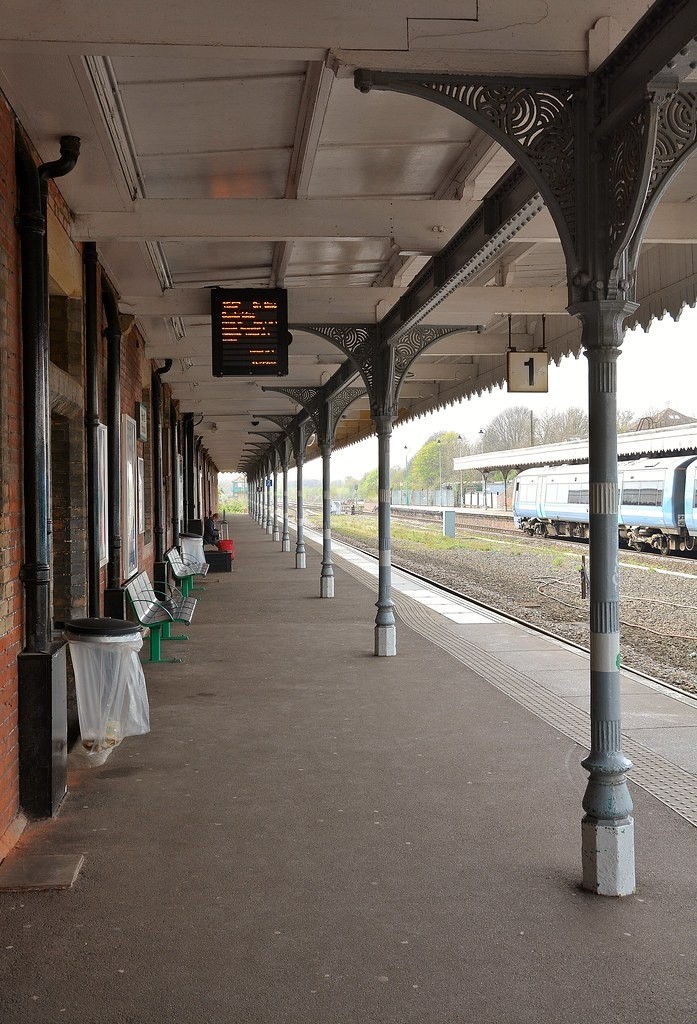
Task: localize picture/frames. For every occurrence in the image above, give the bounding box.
[136,402,147,442]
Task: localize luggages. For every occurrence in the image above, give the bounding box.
[217,521,236,562]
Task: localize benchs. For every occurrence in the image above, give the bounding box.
[165,545,210,599]
[123,570,197,663]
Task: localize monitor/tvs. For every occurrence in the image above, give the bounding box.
[210,287,288,378]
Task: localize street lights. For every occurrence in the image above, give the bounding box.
[404,446,408,504]
[437,440,442,508]
[457,435,464,508]
[479,427,487,509]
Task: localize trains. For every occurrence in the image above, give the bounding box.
[510,455,697,557]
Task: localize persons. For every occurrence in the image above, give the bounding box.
[205,513,220,545]
